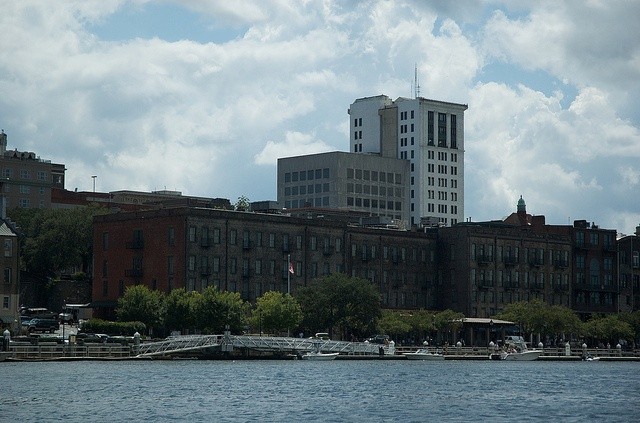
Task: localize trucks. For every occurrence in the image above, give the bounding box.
[59,303,93,323]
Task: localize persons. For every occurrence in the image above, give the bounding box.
[456,340,462,348]
[606,342,611,349]
[364,340,370,344]
[384,337,388,345]
[390,340,395,346]
[616,343,622,349]
[581,342,588,348]
[422,340,429,347]
[488,340,494,347]
[538,340,543,348]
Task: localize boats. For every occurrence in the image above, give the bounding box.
[302,349,338,359]
[405,346,444,357]
[499,348,542,360]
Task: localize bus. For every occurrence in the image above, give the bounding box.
[314,332,331,338]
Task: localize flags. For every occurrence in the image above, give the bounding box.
[289,261,295,274]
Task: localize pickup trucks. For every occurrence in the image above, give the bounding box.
[20,307,58,319]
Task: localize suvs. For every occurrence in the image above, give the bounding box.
[368,333,390,342]
[498,335,524,343]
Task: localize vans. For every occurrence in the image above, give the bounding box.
[21,318,60,332]
